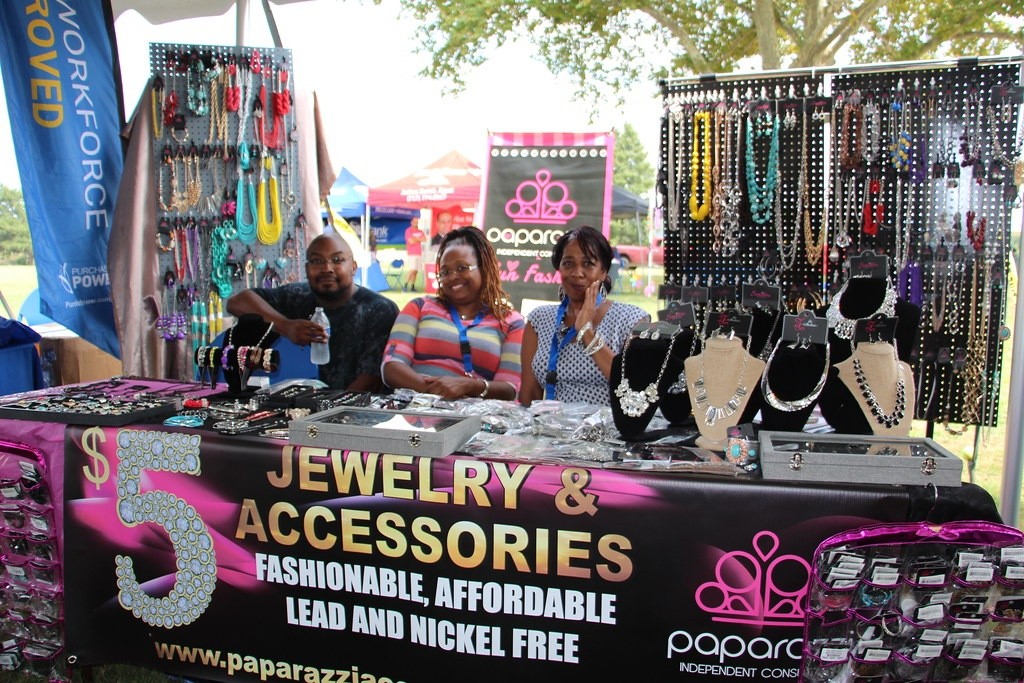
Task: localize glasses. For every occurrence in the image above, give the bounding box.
[438,265,479,280]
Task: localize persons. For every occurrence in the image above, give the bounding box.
[228,233,398,392]
[383,226,525,398]
[519,226,650,404]
[402,216,427,292]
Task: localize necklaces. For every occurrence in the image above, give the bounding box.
[827,276,897,339]
[762,312,782,349]
[148,59,224,383]
[760,337,829,411]
[460,313,476,319]
[667,89,1020,272]
[206,52,308,345]
[903,263,1006,448]
[669,321,702,394]
[692,338,752,425]
[851,349,907,428]
[615,333,676,417]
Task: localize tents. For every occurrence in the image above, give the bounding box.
[322,149,651,249]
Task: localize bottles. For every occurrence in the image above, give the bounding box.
[311,307,330,365]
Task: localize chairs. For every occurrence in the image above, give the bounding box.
[384,259,405,289]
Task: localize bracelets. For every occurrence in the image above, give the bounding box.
[198,346,275,373]
[576,322,603,357]
[477,380,489,398]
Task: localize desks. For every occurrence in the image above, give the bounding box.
[0,377,1005,683]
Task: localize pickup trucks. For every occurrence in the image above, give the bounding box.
[616,237,663,270]
